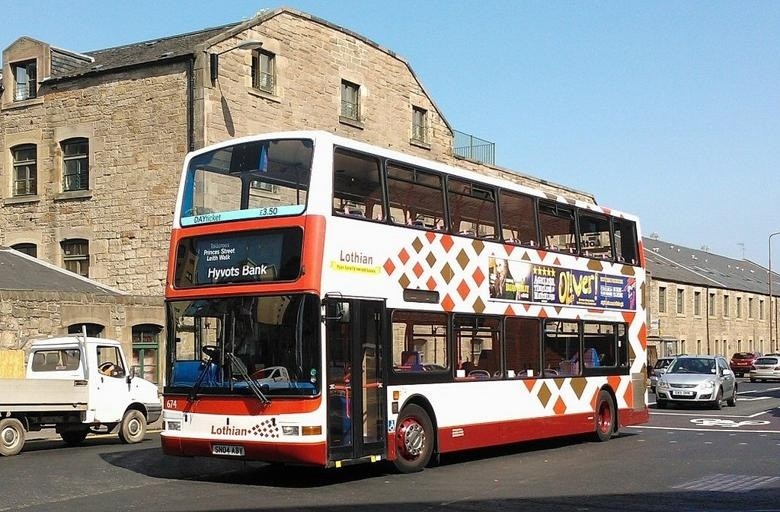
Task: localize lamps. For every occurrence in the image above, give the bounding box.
[210,41,263,79]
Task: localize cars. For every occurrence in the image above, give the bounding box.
[650,356,676,393]
[655,354,738,410]
[749,356,780,383]
[730,351,761,378]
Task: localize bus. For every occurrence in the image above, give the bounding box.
[160,129,651,475]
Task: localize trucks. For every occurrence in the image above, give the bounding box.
[1,325,162,457]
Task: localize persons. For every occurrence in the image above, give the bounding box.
[487,257,516,300]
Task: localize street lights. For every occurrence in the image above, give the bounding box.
[210,39,263,83]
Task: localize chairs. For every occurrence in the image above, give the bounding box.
[394,348,598,379]
[33,353,79,371]
[335,207,605,258]
